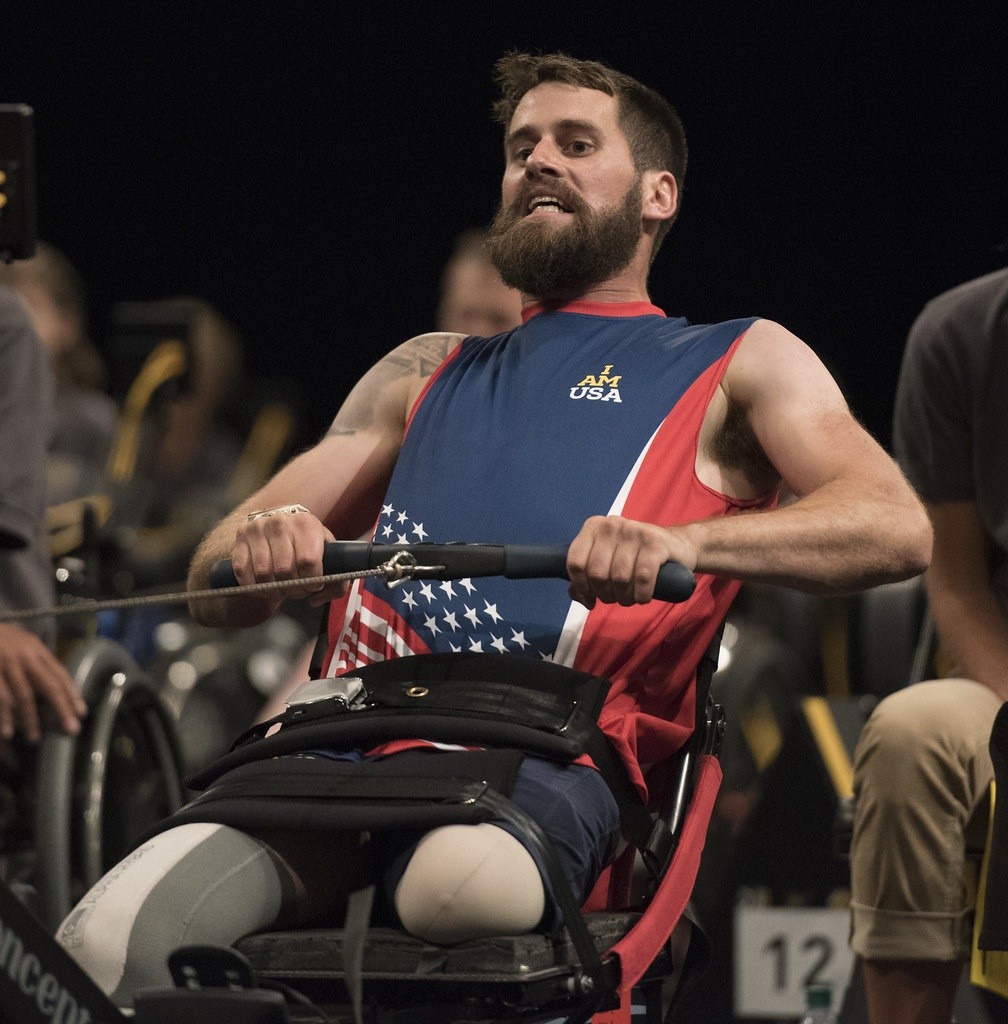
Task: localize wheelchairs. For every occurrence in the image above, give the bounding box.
[0,501,727,1024]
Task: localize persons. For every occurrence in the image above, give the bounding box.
[54,49,938,1022]
[0,202,1007,1023]
[846,269,1008,1024]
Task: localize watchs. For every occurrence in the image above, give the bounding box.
[246,501,311,524]
